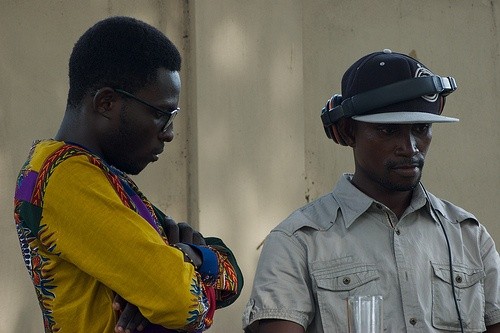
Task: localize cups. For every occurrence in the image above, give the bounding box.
[345,295,383,333]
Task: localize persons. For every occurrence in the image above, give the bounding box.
[244,48,500,333]
[14,17,243,333]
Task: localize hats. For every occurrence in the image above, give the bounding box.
[342,49,461,125]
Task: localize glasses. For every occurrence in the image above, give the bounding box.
[89,84,179,133]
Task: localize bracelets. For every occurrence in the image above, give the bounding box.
[170,244,197,270]
